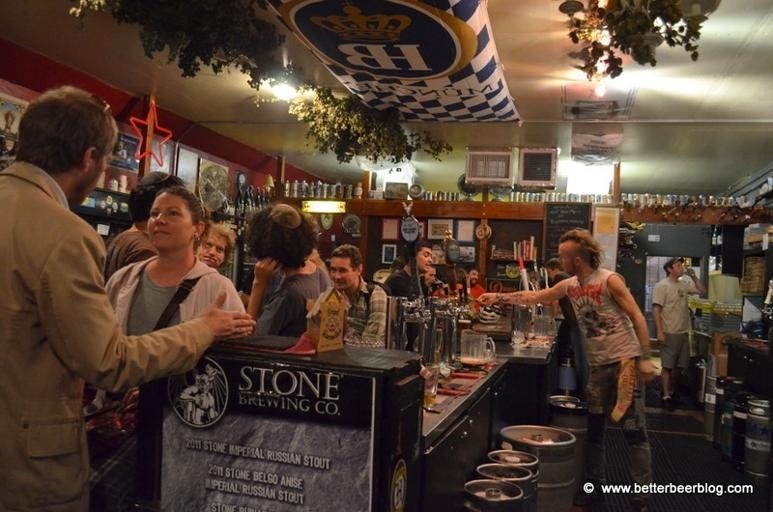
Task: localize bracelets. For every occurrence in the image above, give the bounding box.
[690,273,698,283]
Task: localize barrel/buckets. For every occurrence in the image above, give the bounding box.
[702,375,773,485]
[463,395,589,512]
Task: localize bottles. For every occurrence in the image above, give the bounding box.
[425,190,461,202]
[97,171,128,195]
[237,179,362,216]
[710,224,723,244]
[620,192,746,208]
[510,191,613,205]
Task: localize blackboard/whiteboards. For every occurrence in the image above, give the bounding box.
[542,202,592,264]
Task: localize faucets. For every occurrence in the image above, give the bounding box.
[539,266,549,289]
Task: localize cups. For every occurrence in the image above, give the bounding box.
[460,328,495,363]
[424,362,440,405]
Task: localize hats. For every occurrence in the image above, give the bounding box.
[663,255,685,268]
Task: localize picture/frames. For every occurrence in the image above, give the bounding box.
[381,243,397,265]
[426,218,453,241]
[379,216,400,242]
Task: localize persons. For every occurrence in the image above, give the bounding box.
[544,258,589,397]
[245,203,335,338]
[117,141,126,160]
[198,226,235,267]
[101,170,183,281]
[473,228,655,512]
[651,258,708,401]
[96,187,251,334]
[324,235,486,348]
[0,82,256,511]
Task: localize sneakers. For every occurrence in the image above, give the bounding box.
[661,389,681,412]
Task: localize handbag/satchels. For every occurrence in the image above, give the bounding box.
[86,385,140,441]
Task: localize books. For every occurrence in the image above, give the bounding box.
[513,236,537,262]
